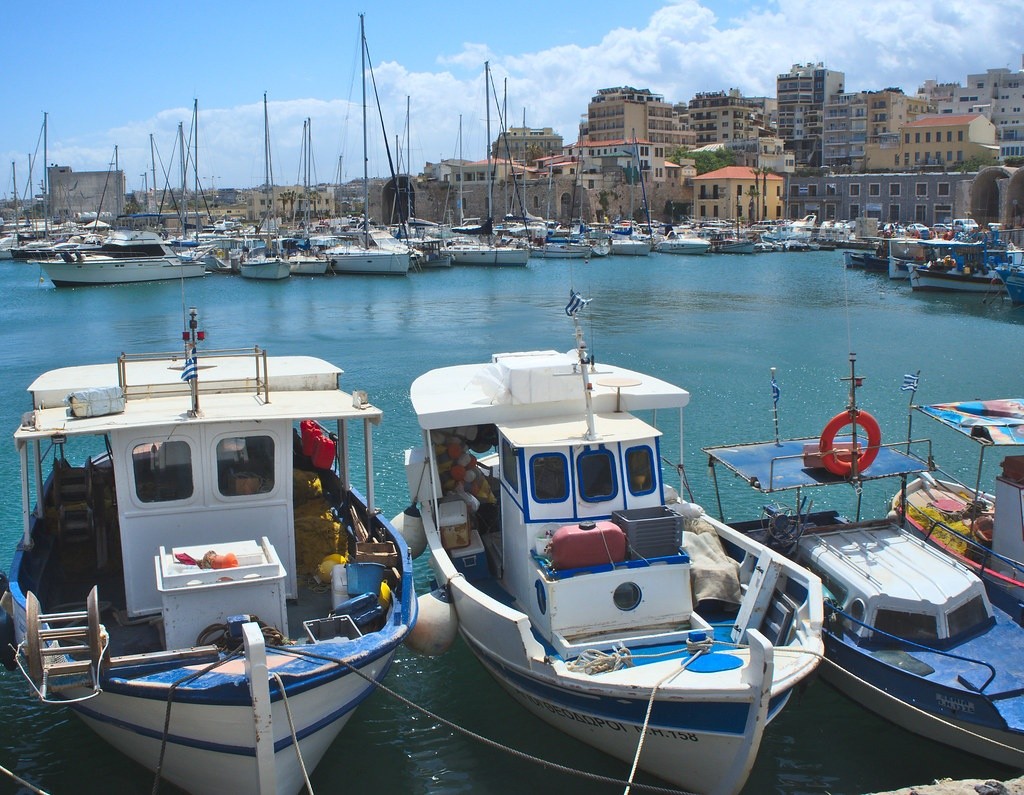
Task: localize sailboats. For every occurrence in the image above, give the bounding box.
[0,10,1024,308]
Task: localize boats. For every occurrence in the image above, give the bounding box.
[404,253,827,795]
[884,398,1023,625]
[0,246,420,795]
[698,256,1023,775]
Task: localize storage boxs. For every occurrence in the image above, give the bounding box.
[437,501,470,549]
[612,505,683,561]
[356,542,399,568]
[449,529,491,580]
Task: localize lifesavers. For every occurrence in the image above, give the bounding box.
[818,409,882,477]
[886,232,891,236]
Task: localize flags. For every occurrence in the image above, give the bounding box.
[769,378,781,402]
[900,374,919,392]
[181,348,198,381]
[565,293,592,316]
